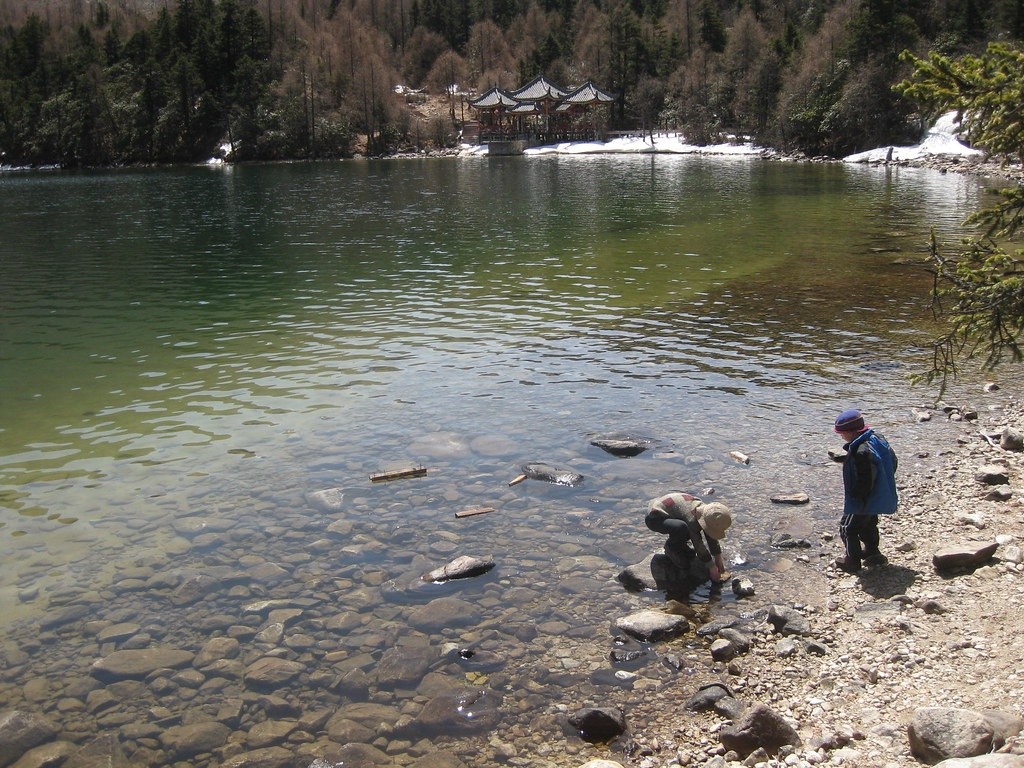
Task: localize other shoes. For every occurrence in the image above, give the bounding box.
[861,548,880,559]
[835,555,861,572]
[664,540,695,571]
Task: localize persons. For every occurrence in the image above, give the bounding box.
[645,492,732,584]
[828,409,898,574]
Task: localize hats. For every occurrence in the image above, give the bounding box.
[695,501,730,539]
[832,411,870,434]
